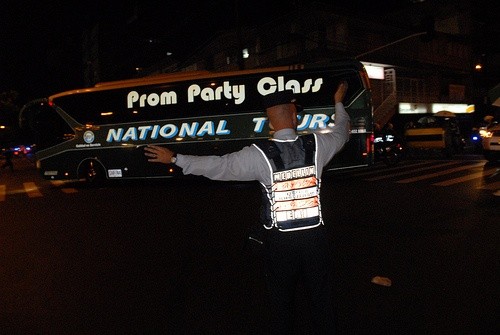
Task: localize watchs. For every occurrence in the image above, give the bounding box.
[170,152,179,165]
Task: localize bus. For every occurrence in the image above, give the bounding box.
[17,61,399,194]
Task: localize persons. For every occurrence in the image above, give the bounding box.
[144,84,351,335]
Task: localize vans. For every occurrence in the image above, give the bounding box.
[486,126,500,153]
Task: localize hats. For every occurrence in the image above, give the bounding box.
[260,89,304,114]
